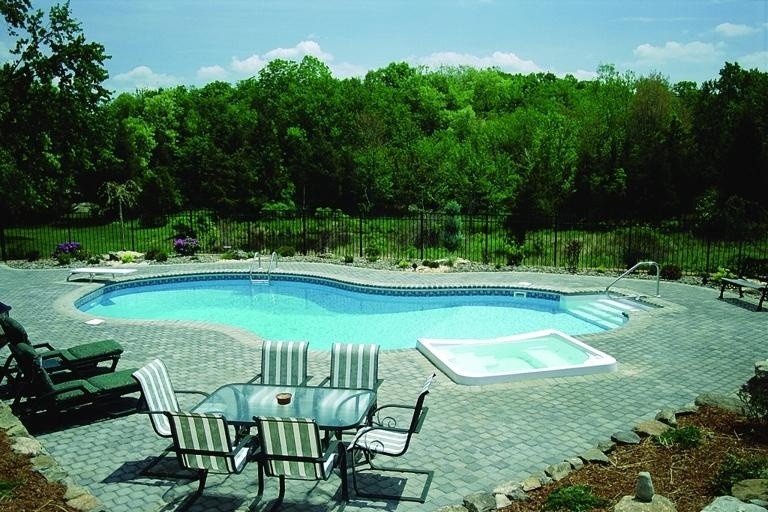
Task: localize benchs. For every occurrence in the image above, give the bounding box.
[716,257,767,311]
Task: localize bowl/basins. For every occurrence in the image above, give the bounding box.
[275,392,292,405]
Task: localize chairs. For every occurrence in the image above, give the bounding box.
[252,414,348,511]
[1,317,123,399]
[131,358,210,480]
[162,410,264,511]
[348,373,437,501]
[190,340,385,493]
[16,343,141,429]
